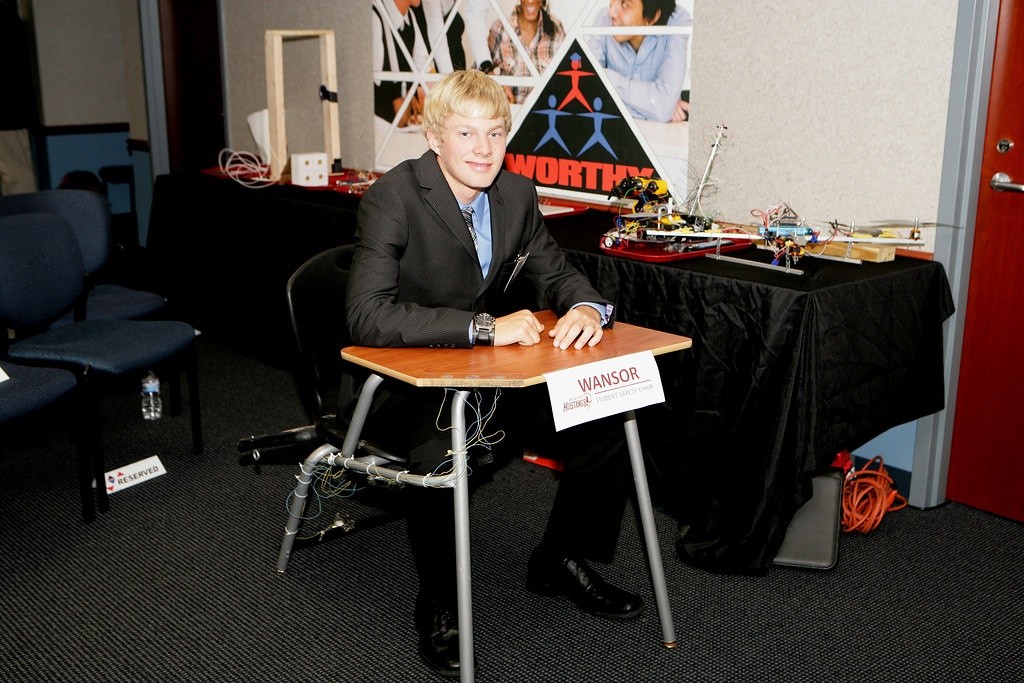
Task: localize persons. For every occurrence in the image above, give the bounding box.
[342,66,646,678]
[586,0,692,124]
[487,0,565,105]
[372,0,515,129]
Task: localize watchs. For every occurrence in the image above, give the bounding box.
[472,313,495,347]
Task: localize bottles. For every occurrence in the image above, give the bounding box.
[141,369,162,420]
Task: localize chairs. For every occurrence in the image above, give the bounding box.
[0,188,202,524]
[236,245,408,462]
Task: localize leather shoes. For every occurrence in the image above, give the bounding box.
[526,545,644,619]
[414,593,479,677]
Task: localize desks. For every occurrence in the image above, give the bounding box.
[192,166,954,579]
[277,310,693,683]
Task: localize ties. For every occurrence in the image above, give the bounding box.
[460,206,478,252]
